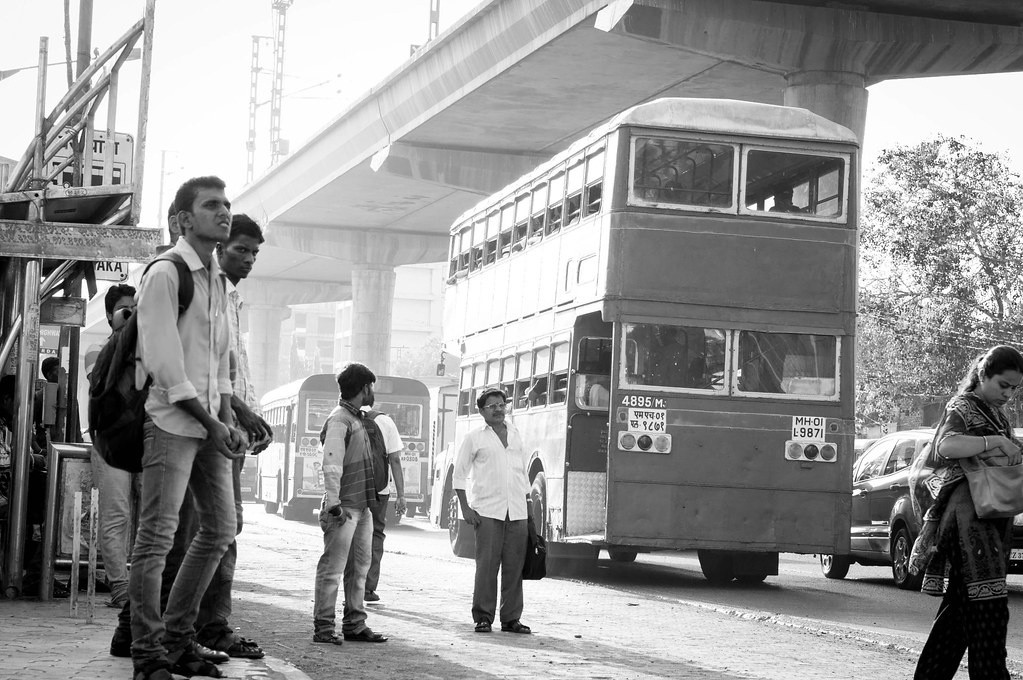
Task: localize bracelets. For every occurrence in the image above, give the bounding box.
[983,436,988,451]
[30,454,35,467]
[39,449,42,453]
[333,506,343,518]
[527,498,533,503]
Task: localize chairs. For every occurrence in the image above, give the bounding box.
[905,447,915,465]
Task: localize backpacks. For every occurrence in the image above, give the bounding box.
[88,249,194,474]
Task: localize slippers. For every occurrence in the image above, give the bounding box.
[53,586,69,597]
[214,633,264,659]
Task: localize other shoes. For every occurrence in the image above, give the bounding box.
[110,634,132,656]
[194,640,229,662]
[132,663,171,680]
[171,649,227,679]
[365,590,380,600]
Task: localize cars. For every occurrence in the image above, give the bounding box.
[819,427,1022,592]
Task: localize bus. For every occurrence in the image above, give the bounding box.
[240,373,430,525]
[431,94,862,585]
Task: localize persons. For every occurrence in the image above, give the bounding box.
[313,363,388,644]
[453,388,536,634]
[769,181,807,213]
[0,357,112,599]
[914,345,1023,680]
[85,177,273,680]
[358,387,407,602]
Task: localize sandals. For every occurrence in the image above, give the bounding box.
[476,619,492,632]
[501,619,530,634]
[315,633,342,647]
[344,628,388,641]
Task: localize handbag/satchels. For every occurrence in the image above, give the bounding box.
[521,515,546,581]
[961,396,1022,518]
